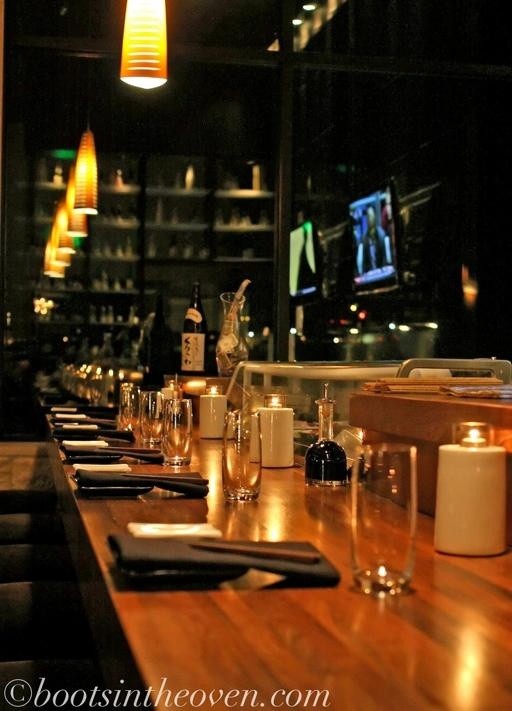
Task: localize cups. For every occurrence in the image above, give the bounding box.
[35,363,506,595]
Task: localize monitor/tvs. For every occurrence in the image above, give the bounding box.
[289,219,319,306]
[347,178,403,296]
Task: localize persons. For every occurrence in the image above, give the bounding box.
[136,293,180,364]
[355,199,392,274]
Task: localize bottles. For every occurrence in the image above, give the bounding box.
[179,281,212,378]
[142,163,278,261]
[34,150,143,364]
[216,292,252,377]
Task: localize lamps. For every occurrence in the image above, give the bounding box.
[118,0,169,89]
[40,0,99,279]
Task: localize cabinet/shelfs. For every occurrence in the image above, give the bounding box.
[0,133,277,361]
